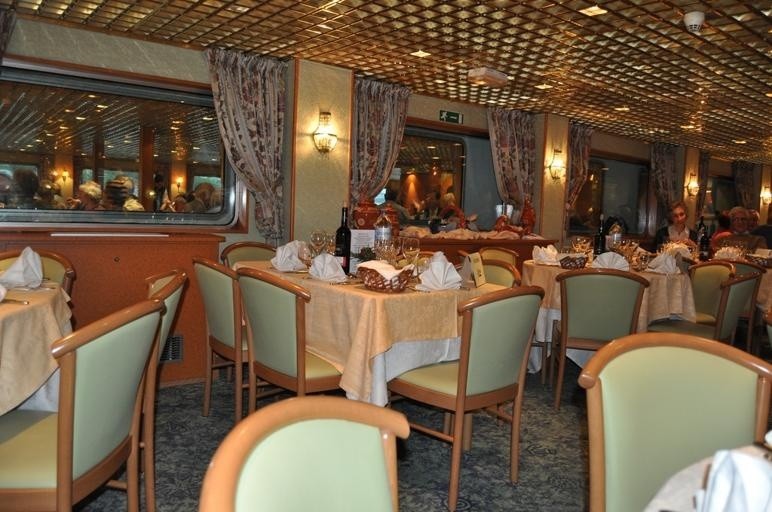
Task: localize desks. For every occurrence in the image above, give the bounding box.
[0,270,61,322]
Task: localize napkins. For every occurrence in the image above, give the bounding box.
[309,253,346,282]
[3,247,43,290]
[270,239,308,272]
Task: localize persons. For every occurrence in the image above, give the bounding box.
[708,202,772,253]
[0,174,223,214]
[377,191,442,225]
[651,200,696,253]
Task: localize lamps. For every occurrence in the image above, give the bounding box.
[311,106,338,155]
[761,186,771,206]
[62,170,69,185]
[177,176,182,191]
[686,172,701,196]
[549,148,566,181]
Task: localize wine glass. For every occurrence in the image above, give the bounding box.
[376,239,433,289]
[712,239,751,259]
[556,233,640,264]
[299,230,335,277]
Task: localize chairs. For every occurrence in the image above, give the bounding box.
[394,225,772,384]
[0,249,74,296]
[552,268,652,409]
[220,241,277,269]
[578,332,772,511]
[199,395,411,511]
[0,298,163,512]
[237,267,342,415]
[191,258,269,424]
[386,285,545,512]
[103,268,188,512]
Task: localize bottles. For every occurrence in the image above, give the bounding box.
[697,216,706,259]
[699,231,710,268]
[336,206,352,277]
[608,217,624,266]
[376,210,394,279]
[639,255,648,273]
[595,215,606,266]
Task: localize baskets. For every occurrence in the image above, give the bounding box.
[560,251,589,269]
[358,264,415,293]
[746,255,772,268]
